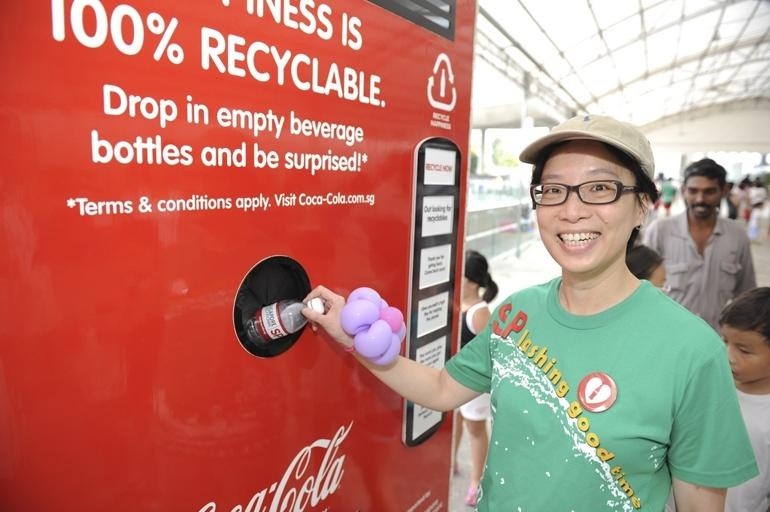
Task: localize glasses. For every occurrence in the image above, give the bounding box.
[530,179,639,207]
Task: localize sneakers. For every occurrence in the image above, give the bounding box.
[464,488,479,506]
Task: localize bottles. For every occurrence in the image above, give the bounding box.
[245,297,324,344]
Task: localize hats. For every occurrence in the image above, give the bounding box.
[518,112,656,183]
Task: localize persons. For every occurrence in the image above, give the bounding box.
[628,114,769,510]
[448,246,500,503]
[300,116,759,511]
